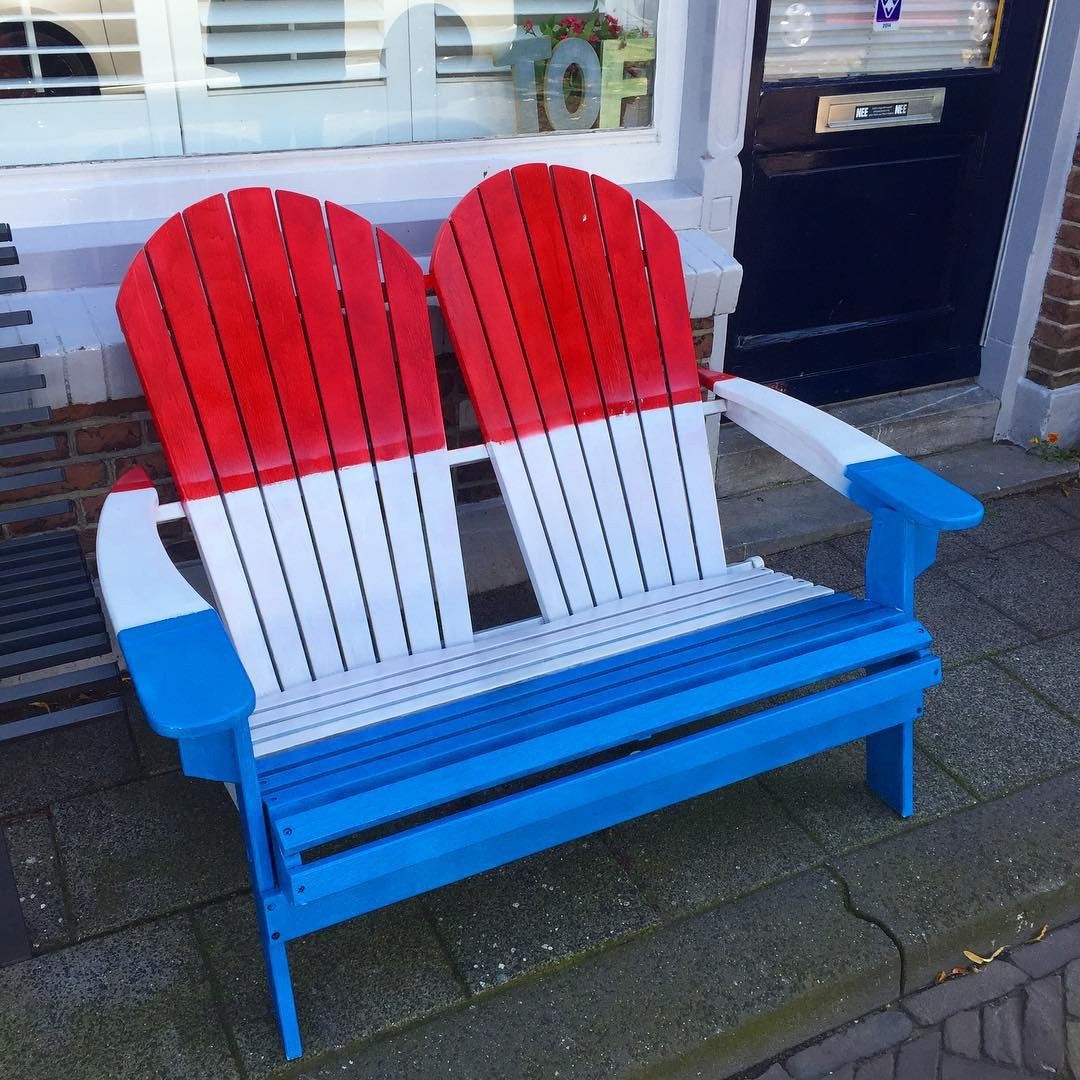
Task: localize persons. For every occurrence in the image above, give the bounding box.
[205,0,345,147]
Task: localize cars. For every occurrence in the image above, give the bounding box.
[1,0,519,99]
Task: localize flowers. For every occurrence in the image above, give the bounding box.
[519,1,649,98]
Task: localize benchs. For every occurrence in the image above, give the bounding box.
[95,162,984,1060]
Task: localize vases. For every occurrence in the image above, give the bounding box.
[535,95,624,133]
